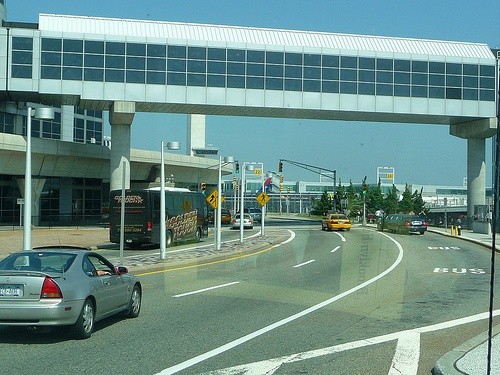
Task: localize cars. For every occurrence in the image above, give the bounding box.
[377,214,428,235]
[0,246,143,339]
[208,208,231,225]
[322,214,352,231]
[231,213,254,230]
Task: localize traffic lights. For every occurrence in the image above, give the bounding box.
[280,183,284,191]
[287,187,290,193]
[281,176,283,183]
[279,162,283,172]
[201,183,206,192]
[235,163,239,173]
[236,185,238,191]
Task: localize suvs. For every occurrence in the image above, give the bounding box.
[237,207,262,224]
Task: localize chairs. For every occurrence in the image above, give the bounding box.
[21,256,42,271]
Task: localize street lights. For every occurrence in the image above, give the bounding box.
[160,140,181,258]
[22,106,54,250]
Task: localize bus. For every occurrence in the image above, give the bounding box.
[109,186,207,250]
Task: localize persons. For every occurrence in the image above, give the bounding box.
[419,210,426,216]
[375,210,381,224]
[409,211,415,216]
[97,270,112,276]
[486,212,489,222]
[489,211,492,224]
